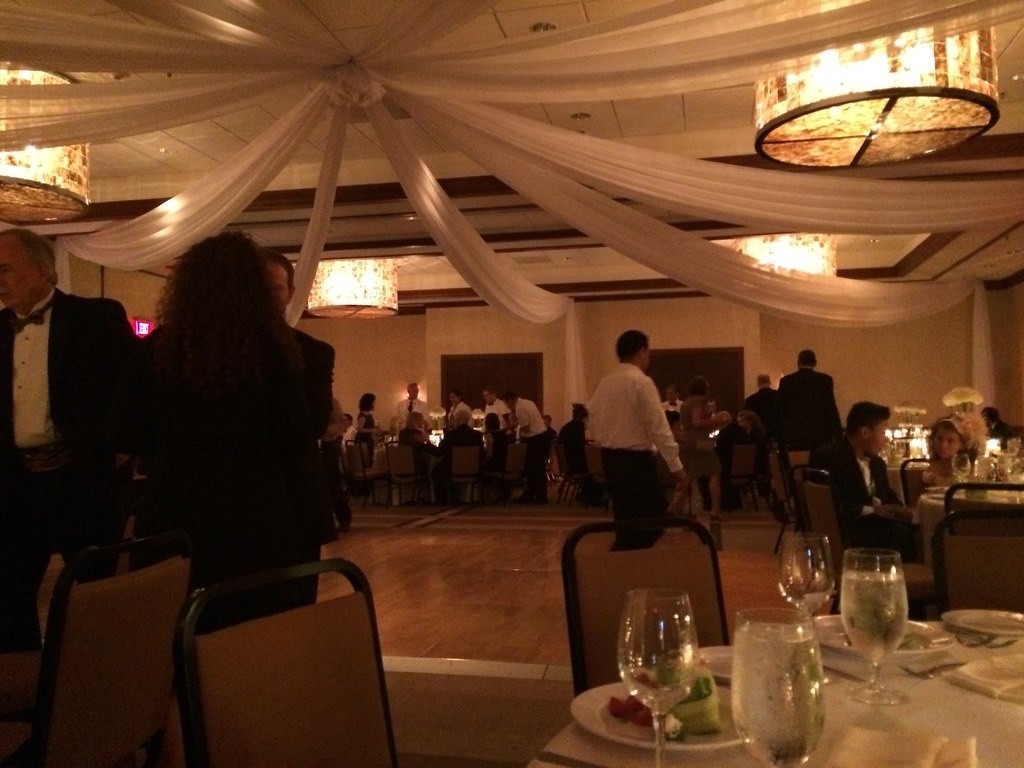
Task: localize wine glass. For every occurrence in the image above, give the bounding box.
[777,533,834,686]
[840,548,910,706]
[880,441,927,468]
[616,588,699,768]
[951,451,1022,502]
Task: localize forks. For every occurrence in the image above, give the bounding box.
[896,662,965,677]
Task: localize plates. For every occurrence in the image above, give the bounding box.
[570,682,743,749]
[941,609,1024,636]
[700,645,733,679]
[813,614,956,652]
[924,486,950,492]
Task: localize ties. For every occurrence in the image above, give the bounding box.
[409,402,413,412]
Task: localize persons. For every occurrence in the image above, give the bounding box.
[0,229,144,651]
[588,329,685,552]
[116,231,591,768]
[659,350,1024,623]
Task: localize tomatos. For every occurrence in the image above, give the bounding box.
[610,696,652,726]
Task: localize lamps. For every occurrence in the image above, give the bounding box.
[307,257,399,319]
[0,60,89,225]
[733,231,839,281]
[753,0,1001,169]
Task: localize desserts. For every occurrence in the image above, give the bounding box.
[657,660,721,736]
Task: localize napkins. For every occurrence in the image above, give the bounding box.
[824,726,978,768]
[949,650,1024,702]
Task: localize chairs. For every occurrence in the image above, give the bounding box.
[0,415,1024,768]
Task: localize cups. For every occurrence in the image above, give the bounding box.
[730,609,824,768]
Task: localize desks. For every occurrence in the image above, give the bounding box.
[887,460,929,504]
[916,490,1024,568]
[527,621,1024,768]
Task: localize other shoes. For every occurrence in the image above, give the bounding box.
[711,512,728,522]
[511,494,546,504]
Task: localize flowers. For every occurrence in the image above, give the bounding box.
[892,404,927,426]
[941,386,984,415]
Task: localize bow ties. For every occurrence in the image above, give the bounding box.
[668,402,677,407]
[9,300,52,333]
